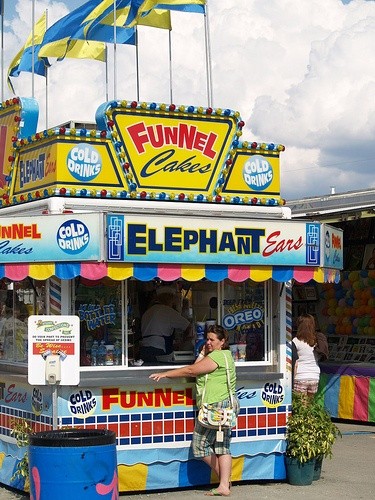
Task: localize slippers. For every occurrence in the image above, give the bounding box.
[205,488,229,496]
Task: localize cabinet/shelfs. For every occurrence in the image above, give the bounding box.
[102,325,135,366]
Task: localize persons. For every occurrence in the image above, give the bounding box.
[365,247,375,270]
[140,287,191,364]
[150,326,238,496]
[1,290,29,322]
[291,313,328,407]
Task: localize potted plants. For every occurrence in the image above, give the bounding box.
[285,391,342,486]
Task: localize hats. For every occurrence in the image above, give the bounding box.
[156,287,176,295]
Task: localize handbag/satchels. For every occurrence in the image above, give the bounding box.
[197,403,234,430]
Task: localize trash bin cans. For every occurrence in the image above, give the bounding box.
[27,428,120,500]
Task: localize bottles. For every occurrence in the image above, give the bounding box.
[90,340,122,366]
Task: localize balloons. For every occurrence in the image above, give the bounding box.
[318,270,375,336]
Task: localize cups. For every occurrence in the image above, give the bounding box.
[193,320,247,362]
